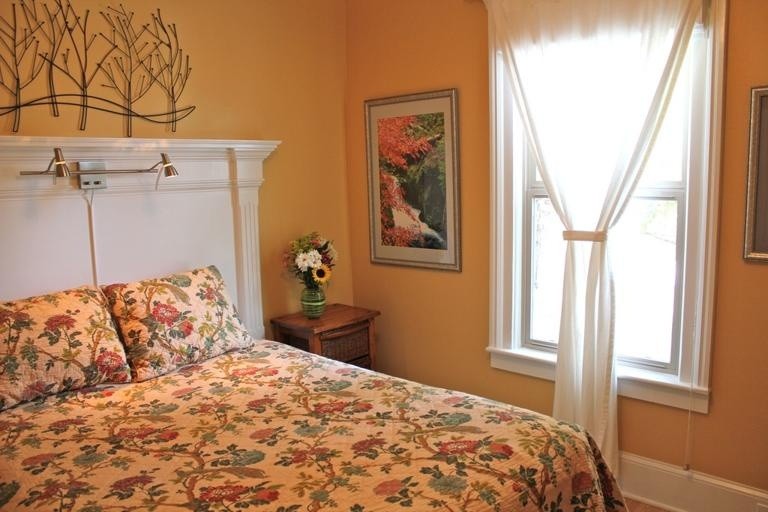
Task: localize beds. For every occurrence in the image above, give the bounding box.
[0,132,626,511]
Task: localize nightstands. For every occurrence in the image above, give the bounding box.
[270,302,381,372]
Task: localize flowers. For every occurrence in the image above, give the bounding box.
[279,231,338,289]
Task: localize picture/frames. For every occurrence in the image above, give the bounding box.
[362,89,462,273]
[742,85,768,261]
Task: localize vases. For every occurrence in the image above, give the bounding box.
[300,287,326,320]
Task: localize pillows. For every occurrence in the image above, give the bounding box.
[0,265,256,412]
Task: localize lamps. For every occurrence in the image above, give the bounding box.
[19,146,180,179]
